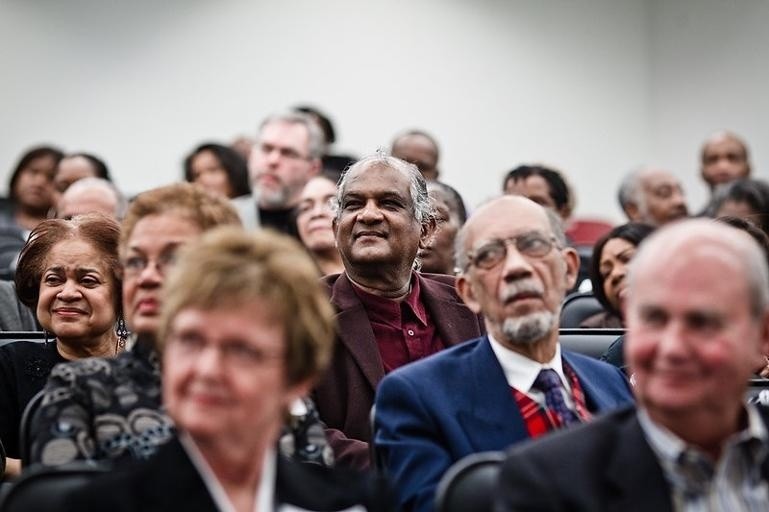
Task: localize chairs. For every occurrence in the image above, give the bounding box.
[1,218,628,512]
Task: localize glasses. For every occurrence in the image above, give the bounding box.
[464,237,567,270]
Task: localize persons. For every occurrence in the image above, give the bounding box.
[1,107,769,512]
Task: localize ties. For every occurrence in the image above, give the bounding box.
[535,369,576,430]
